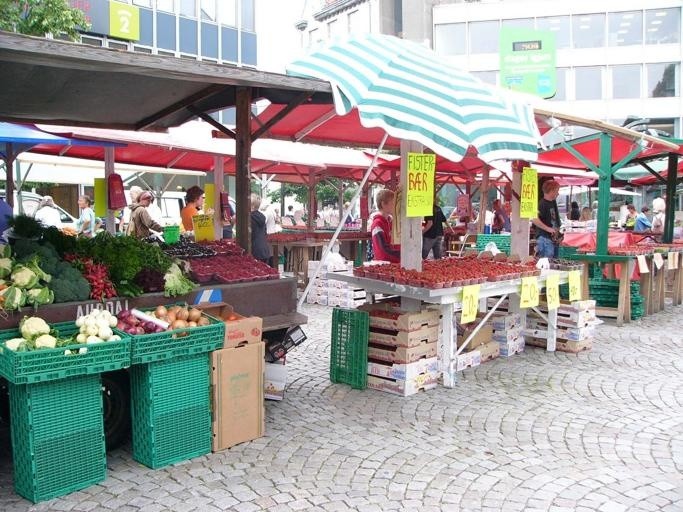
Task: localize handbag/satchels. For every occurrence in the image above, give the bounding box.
[126,220,139,238]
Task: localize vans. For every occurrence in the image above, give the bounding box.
[448,203,480,227]
[117,190,236,235]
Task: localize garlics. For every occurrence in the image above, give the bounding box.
[74,308,122,354]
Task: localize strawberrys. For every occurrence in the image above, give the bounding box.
[352,252,542,290]
[368,301,400,366]
[575,241,683,255]
[189,237,280,284]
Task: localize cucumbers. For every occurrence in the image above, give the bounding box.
[115,279,144,298]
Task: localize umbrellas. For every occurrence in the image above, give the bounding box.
[282,29,539,319]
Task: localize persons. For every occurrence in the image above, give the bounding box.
[571,195,665,234]
[422,196,455,261]
[449,198,510,234]
[33,195,61,228]
[74,183,274,265]
[285,205,294,215]
[531,178,562,258]
[373,187,400,263]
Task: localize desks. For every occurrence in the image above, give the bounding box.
[568,250,683,327]
[269,233,369,280]
[326,265,584,388]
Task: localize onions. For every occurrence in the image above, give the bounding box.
[117,303,210,339]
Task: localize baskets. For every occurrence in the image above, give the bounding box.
[111,301,226,366]
[8,372,110,505]
[329,307,370,390]
[465,233,511,257]
[130,352,212,470]
[1,319,131,386]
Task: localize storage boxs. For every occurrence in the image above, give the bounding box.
[355,303,443,397]
[192,302,264,347]
[209,341,266,454]
[453,310,527,371]
[488,293,604,352]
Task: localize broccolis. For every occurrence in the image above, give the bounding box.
[0,239,92,353]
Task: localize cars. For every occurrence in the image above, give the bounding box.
[0,190,90,237]
[440,207,455,220]
